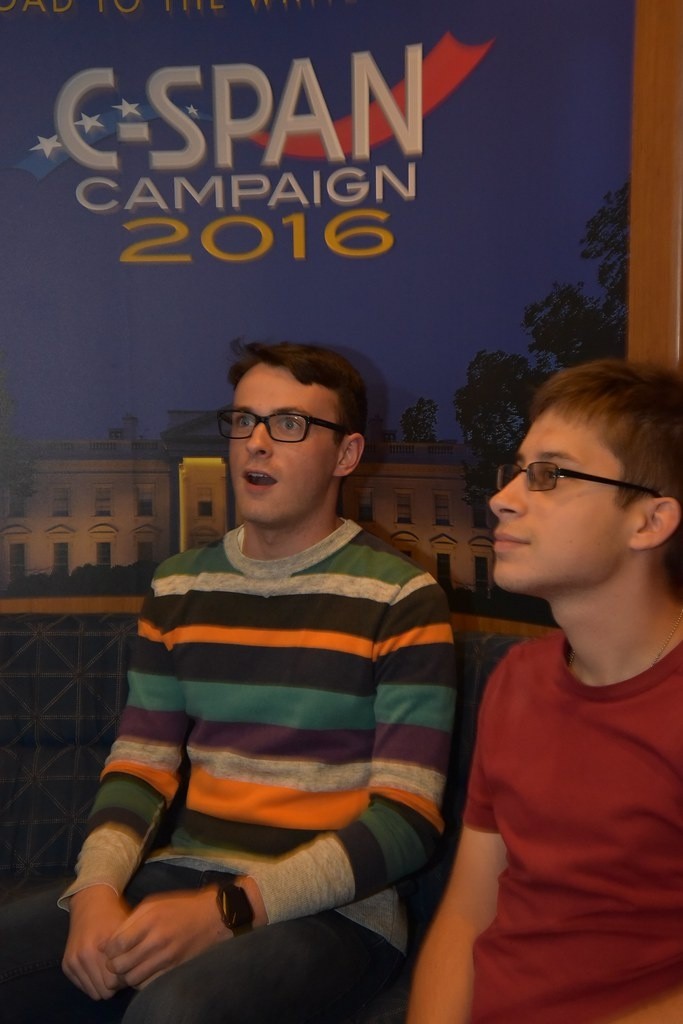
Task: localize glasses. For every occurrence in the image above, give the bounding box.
[497,462,663,499]
[216,409,351,444]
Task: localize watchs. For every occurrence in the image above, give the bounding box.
[216,882,253,936]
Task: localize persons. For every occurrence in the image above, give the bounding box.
[0,342,454,1024]
[408,361,683,1024]
